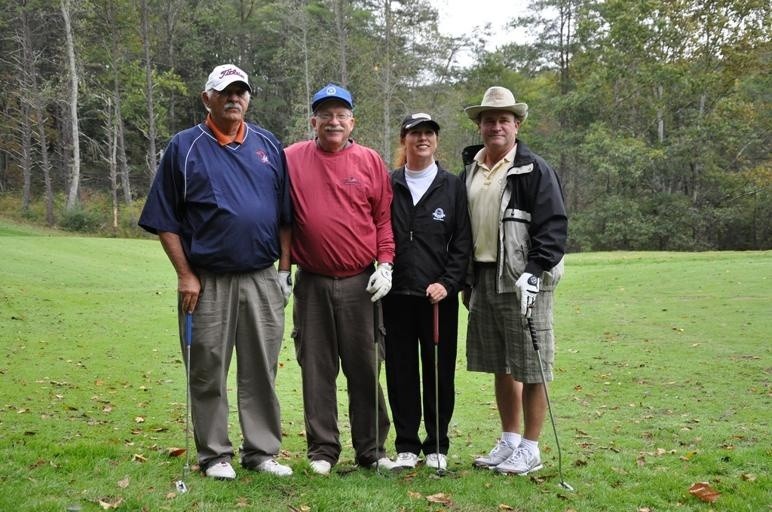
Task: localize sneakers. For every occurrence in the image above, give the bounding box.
[372,452,447,474]
[474,438,516,468]
[307,458,332,478]
[205,459,237,480]
[250,458,294,477]
[494,447,542,474]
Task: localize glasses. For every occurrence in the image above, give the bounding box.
[314,113,350,121]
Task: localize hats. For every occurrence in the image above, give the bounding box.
[465,87,529,126]
[400,112,441,138]
[311,83,354,111]
[204,64,253,94]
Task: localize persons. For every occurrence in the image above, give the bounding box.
[457,84,568,478]
[282,81,404,478]
[381,111,473,472]
[138,63,294,478]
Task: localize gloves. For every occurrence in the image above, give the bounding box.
[365,262,394,303]
[276,270,294,305]
[513,272,540,319]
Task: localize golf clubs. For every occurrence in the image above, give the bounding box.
[371,279,403,478]
[175,311,192,494]
[520,305,574,491]
[433,303,448,476]
